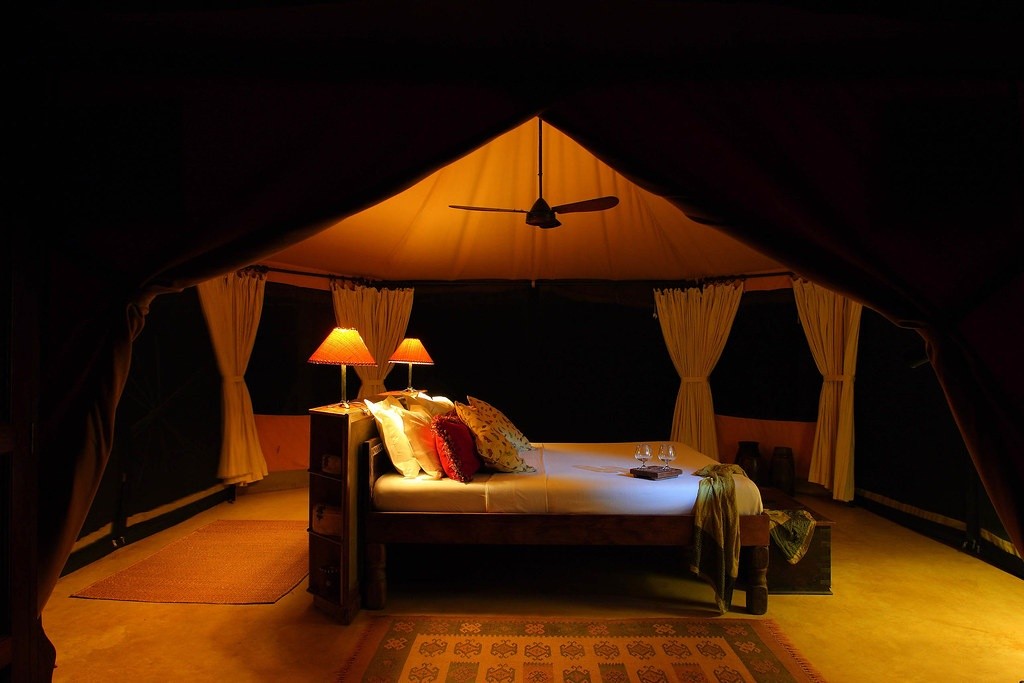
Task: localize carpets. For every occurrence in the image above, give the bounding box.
[70,519,310,605]
[335,610,828,682]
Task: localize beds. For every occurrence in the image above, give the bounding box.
[358,434,770,614]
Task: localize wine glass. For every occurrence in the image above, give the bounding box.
[658,444,677,472]
[634,444,652,470]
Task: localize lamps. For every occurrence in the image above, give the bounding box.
[307,327,378,409]
[387,337,435,393]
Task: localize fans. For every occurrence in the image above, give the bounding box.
[447,118,619,230]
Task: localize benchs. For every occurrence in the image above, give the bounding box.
[759,487,835,596]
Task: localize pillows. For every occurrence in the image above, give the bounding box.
[363,391,539,484]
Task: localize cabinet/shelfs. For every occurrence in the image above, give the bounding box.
[306,388,427,625]
